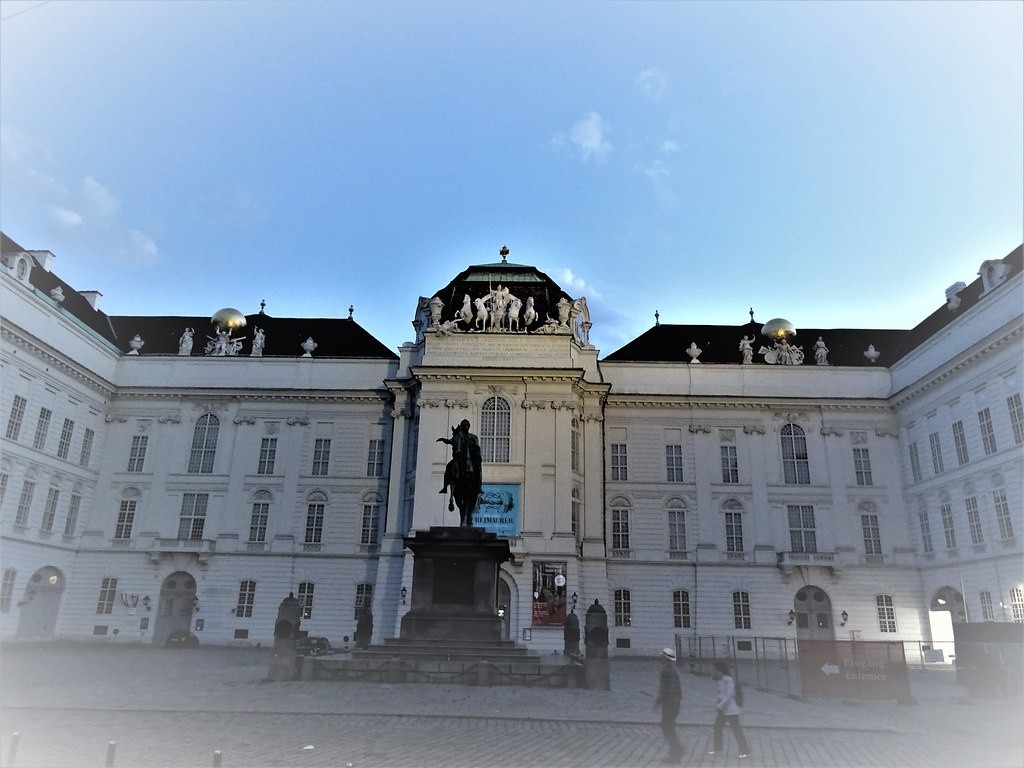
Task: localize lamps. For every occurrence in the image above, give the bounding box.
[787,609,796,626]
[841,611,849,626]
[142,595,152,612]
[401,586,407,604]
[570,592,579,610]
[193,595,199,612]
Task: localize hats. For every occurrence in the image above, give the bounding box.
[661,648,676,661]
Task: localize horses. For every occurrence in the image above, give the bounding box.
[455,295,473,324]
[508,298,522,332]
[448,425,482,528]
[523,297,535,334]
[475,298,490,331]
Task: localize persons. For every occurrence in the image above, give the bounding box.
[490,283,509,311]
[437,420,484,495]
[708,661,750,757]
[774,338,792,364]
[252,325,265,349]
[179,327,195,349]
[653,648,685,763]
[216,325,233,352]
[738,334,755,364]
[813,336,830,365]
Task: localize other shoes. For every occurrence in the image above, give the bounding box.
[663,752,682,764]
[707,752,723,755]
[738,754,751,759]
